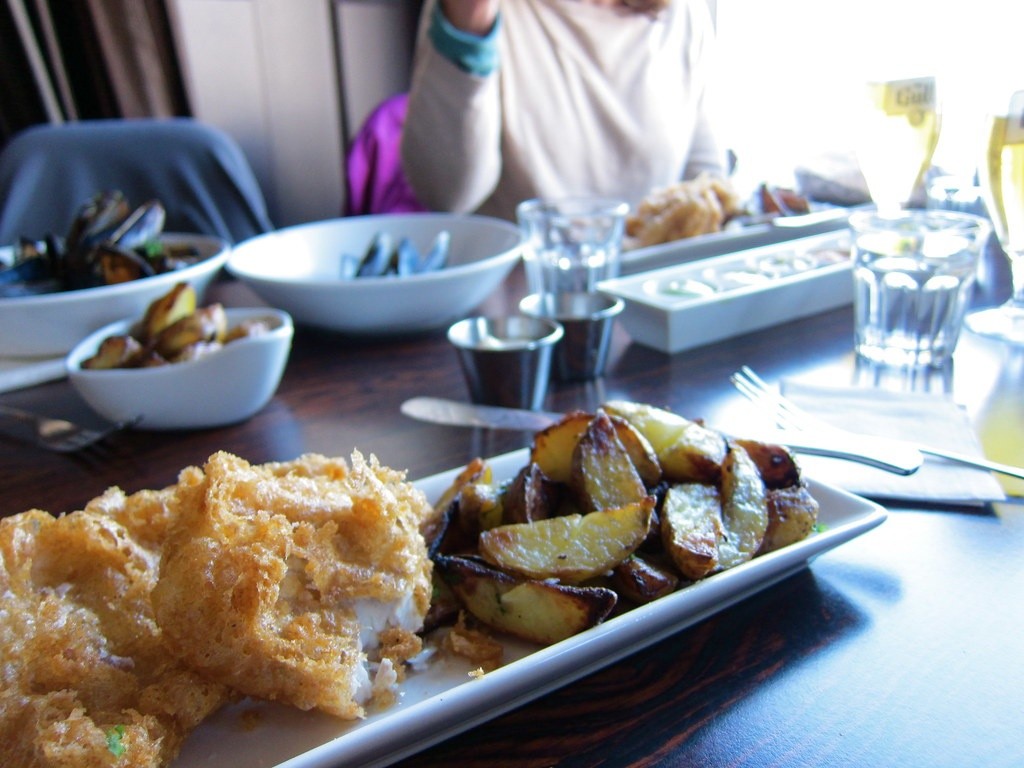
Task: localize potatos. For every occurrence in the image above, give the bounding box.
[422,401,817,642]
[80,282,272,371]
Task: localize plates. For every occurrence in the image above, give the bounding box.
[622,201,836,277]
[166,434,892,768]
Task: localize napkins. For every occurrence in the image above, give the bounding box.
[772,378,1005,507]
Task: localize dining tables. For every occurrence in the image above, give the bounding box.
[0,243,1022,766]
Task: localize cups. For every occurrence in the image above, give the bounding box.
[847,209,992,372]
[519,289,625,384]
[852,84,942,220]
[447,315,564,411]
[514,193,626,297]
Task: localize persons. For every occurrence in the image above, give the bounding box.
[402,0,730,222]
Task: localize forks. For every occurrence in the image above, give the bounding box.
[732,363,1024,480]
[0,404,145,453]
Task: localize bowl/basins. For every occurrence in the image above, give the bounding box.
[599,230,854,354]
[1,234,233,356]
[225,214,525,338]
[65,306,294,431]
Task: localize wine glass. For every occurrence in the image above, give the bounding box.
[966,86,1024,347]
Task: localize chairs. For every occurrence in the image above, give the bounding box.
[344,94,439,216]
[1,118,278,245]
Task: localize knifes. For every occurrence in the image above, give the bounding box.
[401,397,925,475]
